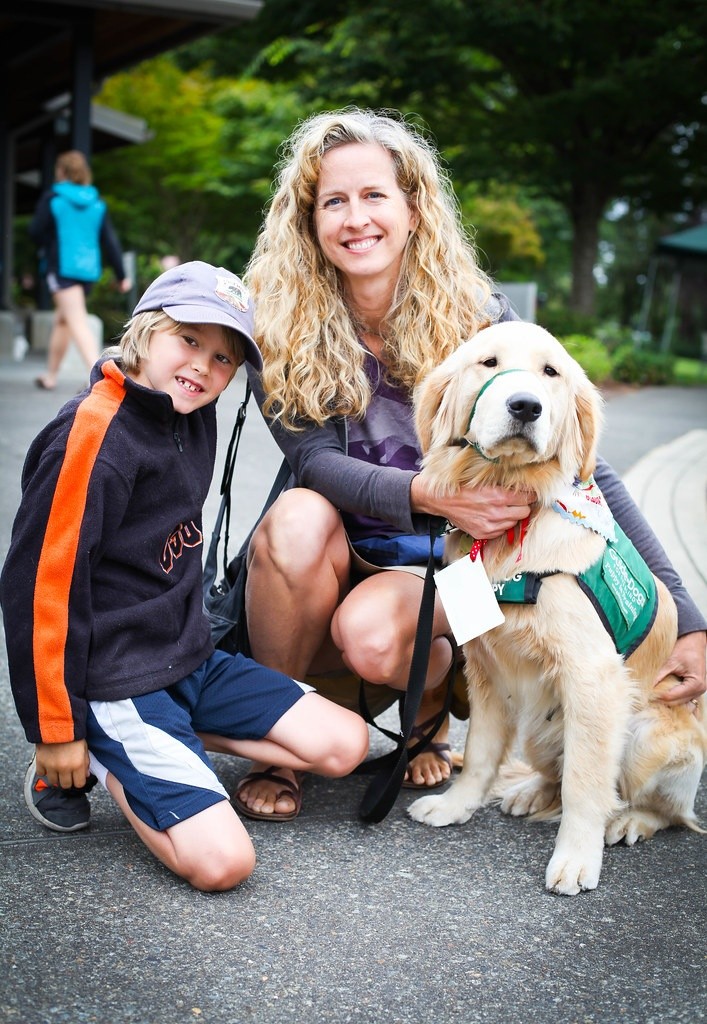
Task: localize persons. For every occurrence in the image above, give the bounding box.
[31,153,134,389]
[0,261,369,892]
[233,115,707,815]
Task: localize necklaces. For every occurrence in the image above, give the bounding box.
[356,318,399,361]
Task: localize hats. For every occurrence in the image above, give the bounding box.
[132,262,264,375]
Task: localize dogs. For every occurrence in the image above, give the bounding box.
[411,322,707,895]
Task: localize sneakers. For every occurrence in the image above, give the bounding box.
[25,747,99,831]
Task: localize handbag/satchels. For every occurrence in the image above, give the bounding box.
[202,543,249,657]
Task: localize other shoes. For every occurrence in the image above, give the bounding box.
[35,374,55,389]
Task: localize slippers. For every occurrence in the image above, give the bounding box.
[399,739,453,789]
[233,759,307,820]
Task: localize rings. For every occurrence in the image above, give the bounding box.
[691,699,697,704]
